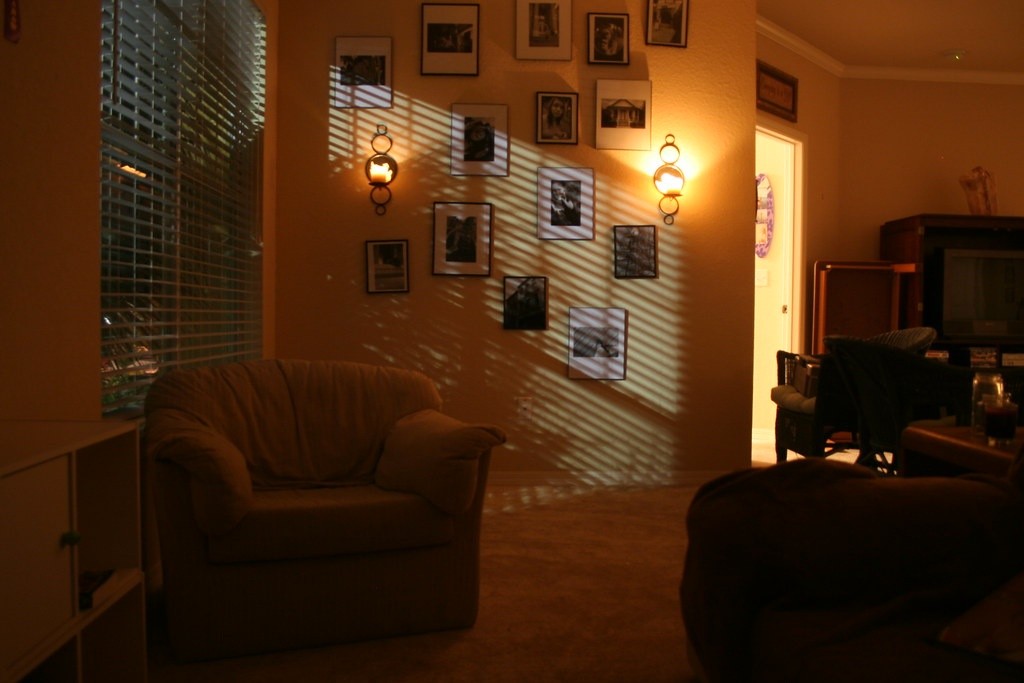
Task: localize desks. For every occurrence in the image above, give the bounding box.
[898,416,1024,479]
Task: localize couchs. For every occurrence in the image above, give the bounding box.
[140,358,506,666]
[677,443,1024,683]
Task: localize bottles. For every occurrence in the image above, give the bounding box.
[972,371,1019,449]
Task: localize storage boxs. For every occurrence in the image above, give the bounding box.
[792,354,821,400]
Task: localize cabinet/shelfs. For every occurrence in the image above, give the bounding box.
[883,213,1024,427]
[0,417,151,683]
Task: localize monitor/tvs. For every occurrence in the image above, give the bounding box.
[925,248,1024,340]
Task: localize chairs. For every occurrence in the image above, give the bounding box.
[772,325,977,470]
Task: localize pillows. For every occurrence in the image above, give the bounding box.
[926,569,1024,673]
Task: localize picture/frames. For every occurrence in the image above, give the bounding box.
[536,168,594,240]
[613,225,657,279]
[449,102,508,178]
[503,276,546,330]
[431,202,492,277]
[334,36,393,108]
[365,239,410,294]
[420,2,480,77]
[536,91,579,145]
[515,0,573,60]
[595,79,652,152]
[586,12,630,66]
[568,306,628,380]
[755,57,798,124]
[645,0,690,48]
